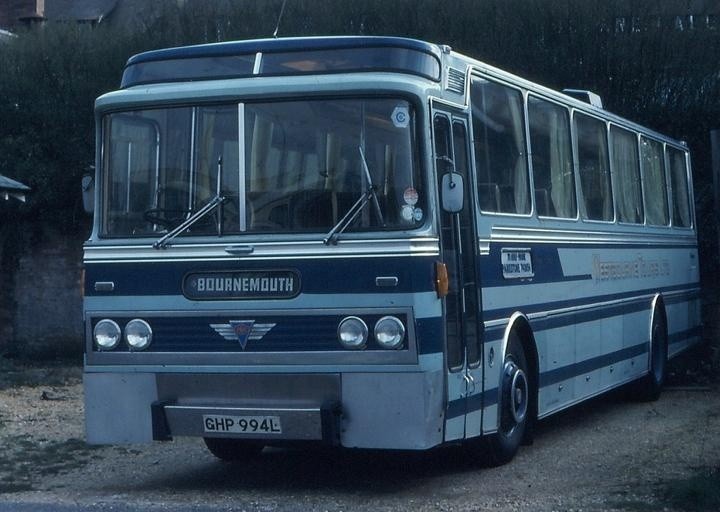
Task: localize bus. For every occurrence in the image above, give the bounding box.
[75,30,704,486]
